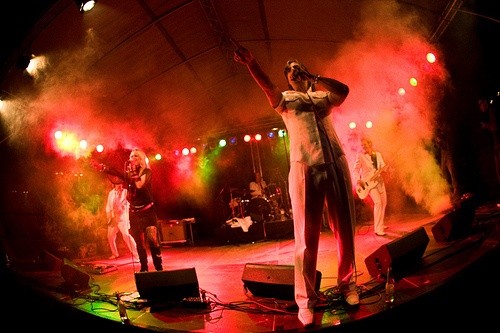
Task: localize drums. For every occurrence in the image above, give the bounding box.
[263,184,281,202]
[236,199,249,217]
[246,197,273,222]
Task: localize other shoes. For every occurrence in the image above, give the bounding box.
[377,231,386,236]
[344,289,360,306]
[135,259,140,263]
[298,308,315,327]
[108,254,119,259]
[383,226,388,230]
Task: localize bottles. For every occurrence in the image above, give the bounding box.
[5,255,10,266]
[385,266,394,303]
[116,293,128,320]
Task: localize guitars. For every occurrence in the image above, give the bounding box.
[356,162,389,200]
[106,198,127,225]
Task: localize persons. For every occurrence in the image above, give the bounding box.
[353,136,389,236]
[248,171,267,197]
[233,47,359,328]
[123,149,163,271]
[105,177,141,262]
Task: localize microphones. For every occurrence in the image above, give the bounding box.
[293,65,300,70]
[126,159,133,162]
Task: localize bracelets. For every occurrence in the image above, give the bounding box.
[314,74,321,83]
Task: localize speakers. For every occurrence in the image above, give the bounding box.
[28,247,62,269]
[134,267,199,298]
[62,258,90,288]
[431,205,476,241]
[159,221,188,241]
[365,226,429,277]
[241,263,322,296]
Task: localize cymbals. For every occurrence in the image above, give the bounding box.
[236,190,255,193]
[215,183,227,200]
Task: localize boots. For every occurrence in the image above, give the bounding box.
[149,245,163,271]
[137,248,148,272]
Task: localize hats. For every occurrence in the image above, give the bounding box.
[107,175,124,184]
